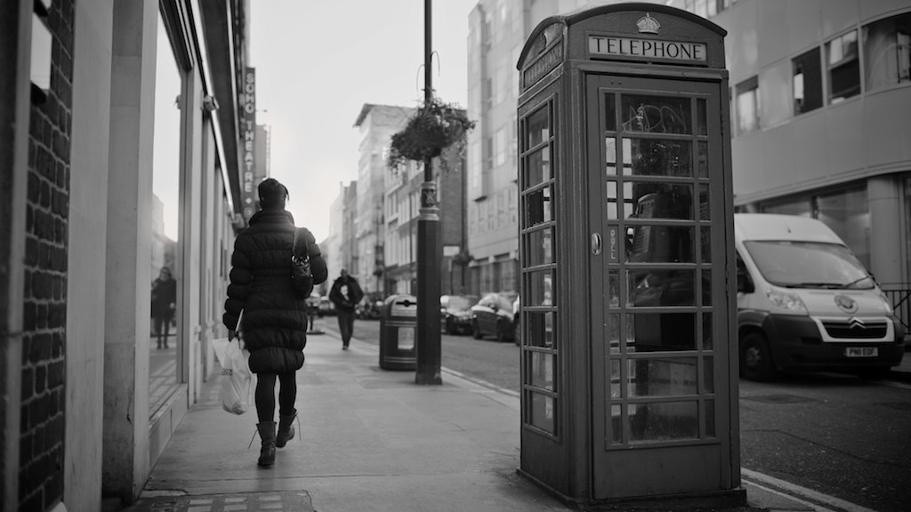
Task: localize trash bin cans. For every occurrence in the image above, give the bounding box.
[380,294,419,372]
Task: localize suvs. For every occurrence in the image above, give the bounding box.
[510,278,557,347]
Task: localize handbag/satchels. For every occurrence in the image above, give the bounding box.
[290,253,315,300]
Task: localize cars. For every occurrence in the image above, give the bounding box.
[437,295,475,337]
[303,291,385,320]
[468,291,513,342]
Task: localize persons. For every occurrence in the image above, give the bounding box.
[222,178,327,468]
[355,298,382,321]
[151,266,176,350]
[329,268,364,352]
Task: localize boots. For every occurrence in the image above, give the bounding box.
[256,421,278,466]
[277,407,297,447]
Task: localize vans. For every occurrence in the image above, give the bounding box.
[732,210,904,385]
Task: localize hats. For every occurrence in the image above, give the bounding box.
[258,178,287,203]
